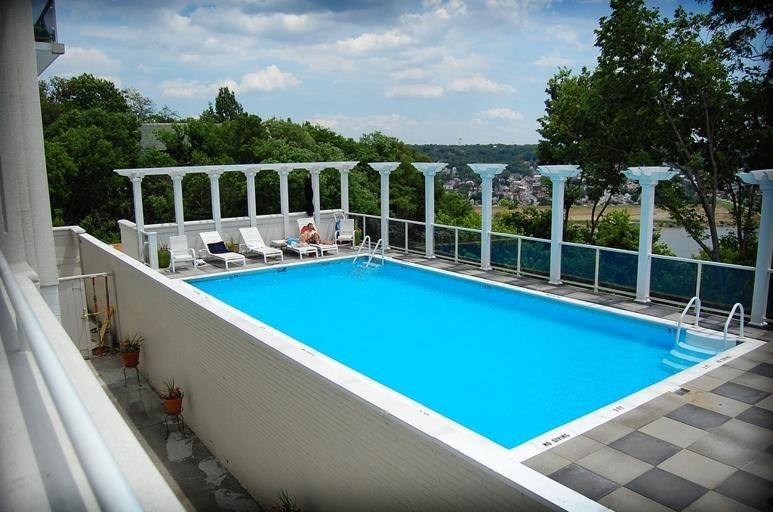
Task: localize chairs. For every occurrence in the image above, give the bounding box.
[240,228,284,264]
[271,239,318,260]
[169,236,199,273]
[335,219,356,251]
[297,217,338,259]
[199,232,246,270]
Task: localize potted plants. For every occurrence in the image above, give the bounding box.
[158,242,171,268]
[158,376,184,415]
[354,219,362,245]
[118,333,144,366]
[226,234,239,253]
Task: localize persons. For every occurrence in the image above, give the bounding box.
[283,234,307,249]
[304,223,335,245]
